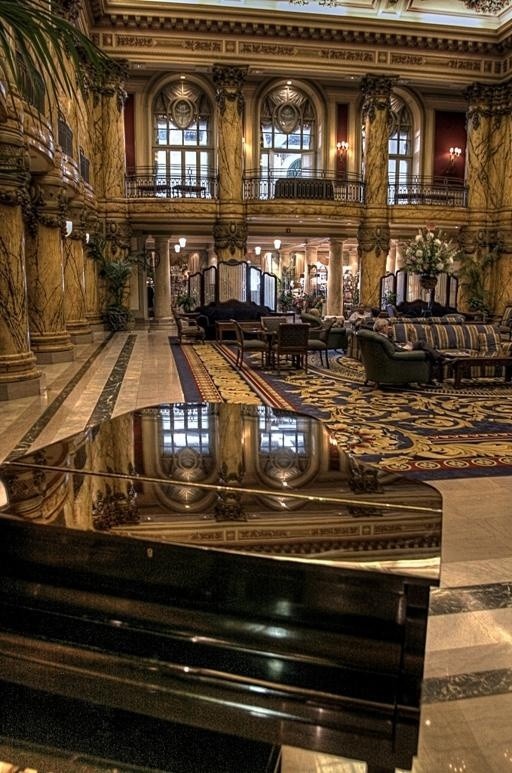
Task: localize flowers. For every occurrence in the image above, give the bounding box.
[397,223,464,274]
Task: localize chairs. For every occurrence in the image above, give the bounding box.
[230,313,348,377]
[171,306,206,345]
[354,329,430,390]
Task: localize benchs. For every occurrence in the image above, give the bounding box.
[394,191,455,206]
[137,183,206,199]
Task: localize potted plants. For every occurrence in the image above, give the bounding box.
[88,236,136,330]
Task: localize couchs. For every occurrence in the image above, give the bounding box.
[184,298,272,340]
[344,305,511,377]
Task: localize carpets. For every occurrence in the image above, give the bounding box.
[167,334,511,482]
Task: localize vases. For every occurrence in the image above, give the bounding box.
[419,275,437,289]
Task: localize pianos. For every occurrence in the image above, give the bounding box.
[0,403,442,773]
